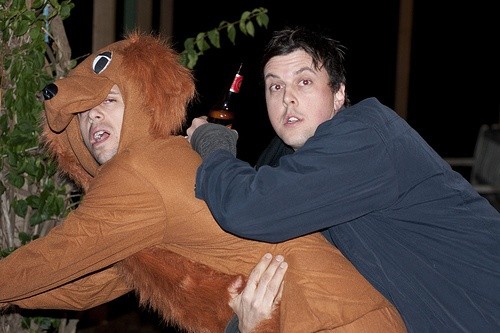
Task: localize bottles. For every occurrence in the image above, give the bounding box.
[207,62,245,129]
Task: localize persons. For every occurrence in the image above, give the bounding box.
[0,26,407,333]
[186,26,500,333]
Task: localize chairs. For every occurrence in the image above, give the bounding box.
[443,124,500,212]
